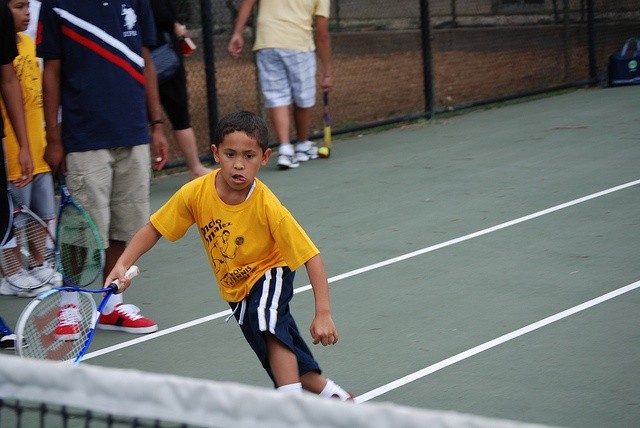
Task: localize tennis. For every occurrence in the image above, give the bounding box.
[318,147,329,158]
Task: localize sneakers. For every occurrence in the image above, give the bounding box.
[28,261,63,285]
[295,142,319,162]
[277,146,300,168]
[97,303,158,334]
[0,318,30,350]
[53,304,80,340]
[0,269,51,298]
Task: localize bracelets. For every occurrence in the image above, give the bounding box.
[150,119,164,127]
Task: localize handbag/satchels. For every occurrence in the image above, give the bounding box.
[151,41,182,78]
[608,37,640,88]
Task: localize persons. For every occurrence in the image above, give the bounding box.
[226,0,333,168]
[146,0,215,181]
[34,2,167,342]
[0,2,62,298]
[0,5,35,351]
[102,112,355,403]
